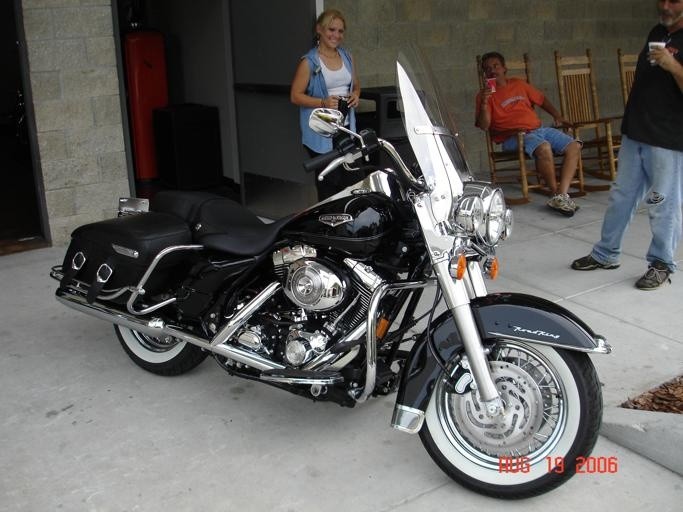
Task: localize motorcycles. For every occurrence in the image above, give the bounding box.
[48,48,614,500]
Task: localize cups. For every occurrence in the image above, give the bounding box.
[337,95,350,126]
[487,78,497,93]
[648,42,666,66]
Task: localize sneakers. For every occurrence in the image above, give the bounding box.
[571,254,621,270]
[545,193,580,217]
[635,259,671,291]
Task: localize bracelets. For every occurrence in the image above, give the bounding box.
[318,97,329,107]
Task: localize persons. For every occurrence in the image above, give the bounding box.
[290,8,362,209]
[570,1,683,289]
[472,50,579,219]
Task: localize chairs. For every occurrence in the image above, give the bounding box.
[555,48,625,182]
[476,52,586,205]
[617,49,640,109]
